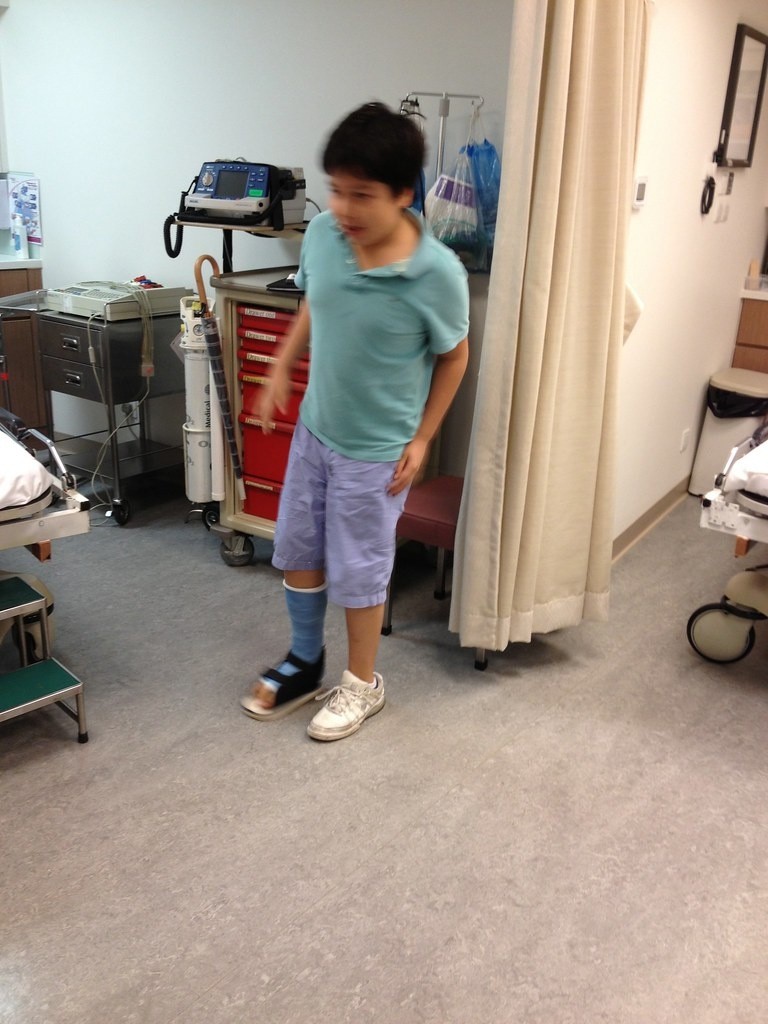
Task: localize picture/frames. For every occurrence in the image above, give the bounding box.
[717,23,768,167]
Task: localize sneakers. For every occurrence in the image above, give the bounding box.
[306,667,386,741]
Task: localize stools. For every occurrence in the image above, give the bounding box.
[381,477,489,671]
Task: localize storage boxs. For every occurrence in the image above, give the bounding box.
[237,304,311,521]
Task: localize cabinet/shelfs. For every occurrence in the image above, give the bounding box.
[731,298,768,375]
[210,264,310,566]
[36,311,185,525]
[0,268,55,450]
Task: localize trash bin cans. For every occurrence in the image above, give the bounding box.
[686,366,768,499]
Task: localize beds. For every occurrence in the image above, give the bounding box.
[0,408,89,563]
[699,421,767,544]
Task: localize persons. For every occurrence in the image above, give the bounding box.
[238,104,471,743]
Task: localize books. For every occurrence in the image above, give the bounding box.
[265,278,304,292]
